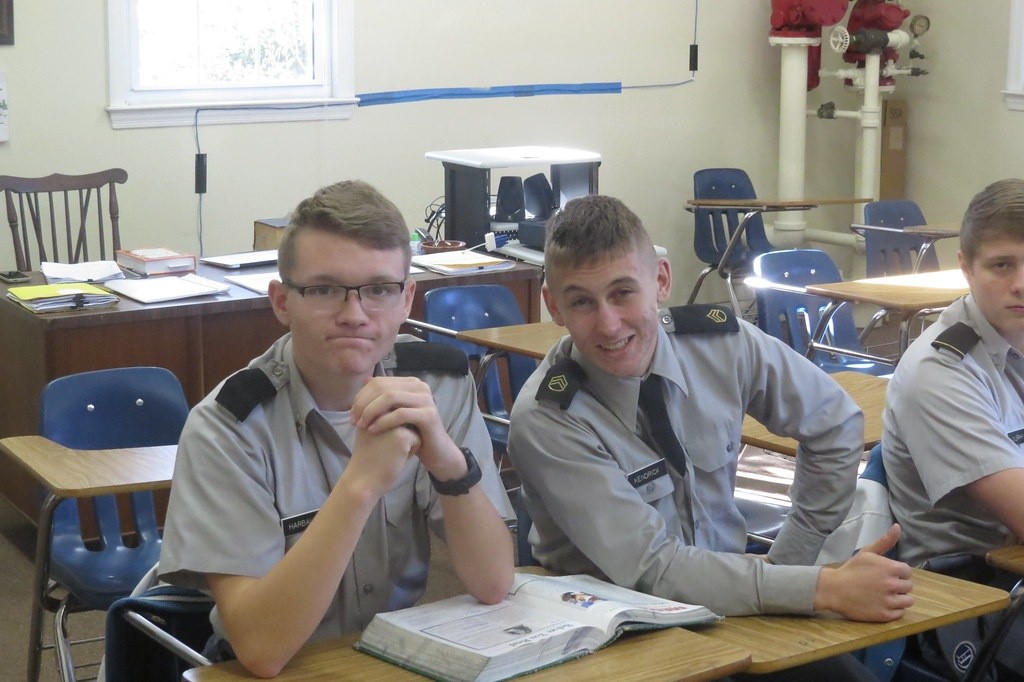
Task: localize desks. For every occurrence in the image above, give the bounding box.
[680,560,1011,674]
[0,435,178,682]
[964,544,1024,682]
[182,566,750,682]
[738,371,890,463]
[688,198,874,275]
[804,270,970,378]
[0,218,541,565]
[429,146,603,248]
[454,321,570,400]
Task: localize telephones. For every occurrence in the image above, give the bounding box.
[414,228,434,242]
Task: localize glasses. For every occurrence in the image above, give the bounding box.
[282,277,407,312]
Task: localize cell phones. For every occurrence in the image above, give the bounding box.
[0,270,32,283]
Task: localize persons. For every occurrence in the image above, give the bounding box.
[882,180,1024,682]
[157,178,517,677]
[506,196,914,682]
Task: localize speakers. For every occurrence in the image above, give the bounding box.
[495,176,525,223]
[524,172,557,220]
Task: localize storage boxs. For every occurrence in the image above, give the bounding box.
[879,98,907,194]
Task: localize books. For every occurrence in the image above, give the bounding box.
[353,572,725,682]
[115,247,197,276]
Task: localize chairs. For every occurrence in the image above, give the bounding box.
[903,225,961,274]
[0,168,1024,682]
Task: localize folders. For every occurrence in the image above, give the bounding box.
[410,249,517,275]
[5,282,120,314]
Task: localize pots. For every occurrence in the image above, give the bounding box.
[415,227,467,255]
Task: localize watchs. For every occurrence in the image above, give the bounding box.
[427,445,482,500]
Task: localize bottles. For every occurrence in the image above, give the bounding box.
[410,233,421,255]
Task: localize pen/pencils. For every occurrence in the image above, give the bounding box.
[90,283,114,293]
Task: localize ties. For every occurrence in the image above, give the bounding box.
[638,373,687,477]
